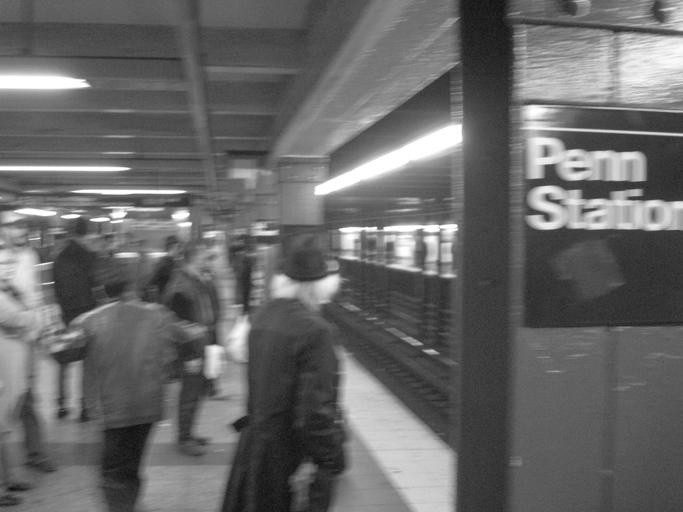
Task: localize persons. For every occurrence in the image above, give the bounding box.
[1,206,253,512]
[222,244,350,510]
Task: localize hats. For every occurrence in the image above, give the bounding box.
[283,246,340,282]
[0,209,29,226]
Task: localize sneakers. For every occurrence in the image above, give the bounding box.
[179,429,214,458]
[58,405,97,422]
[1,453,56,507]
